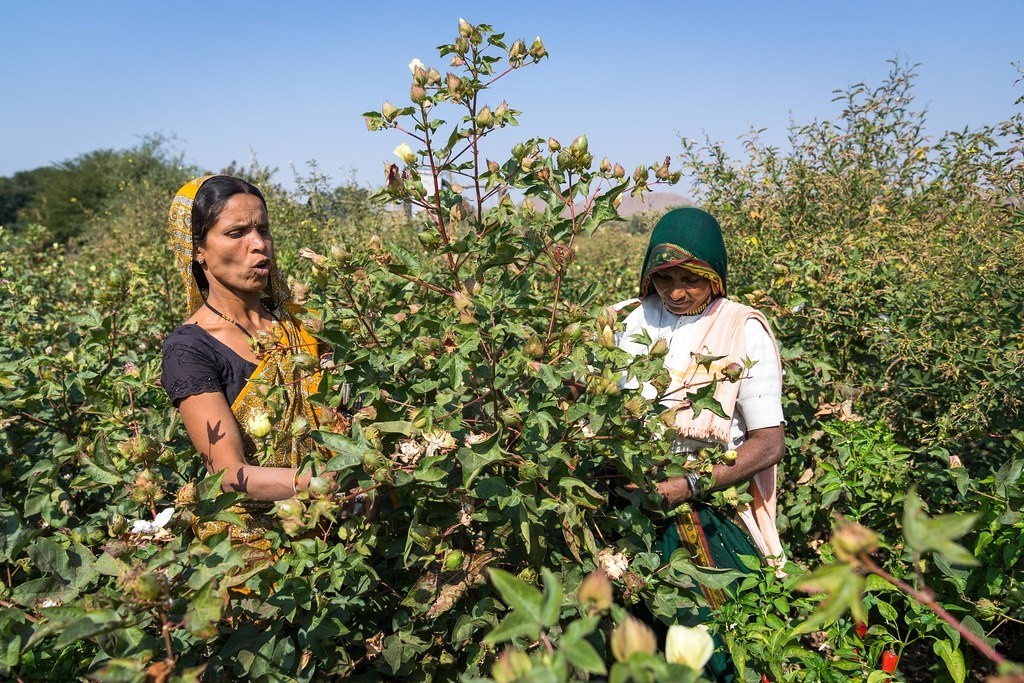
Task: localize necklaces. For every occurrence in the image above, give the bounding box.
[687,294,712,317]
[204,296,289,354]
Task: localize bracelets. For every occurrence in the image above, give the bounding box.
[687,470,702,499]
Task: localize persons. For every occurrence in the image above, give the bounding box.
[605,206,802,683]
[158,176,364,594]
